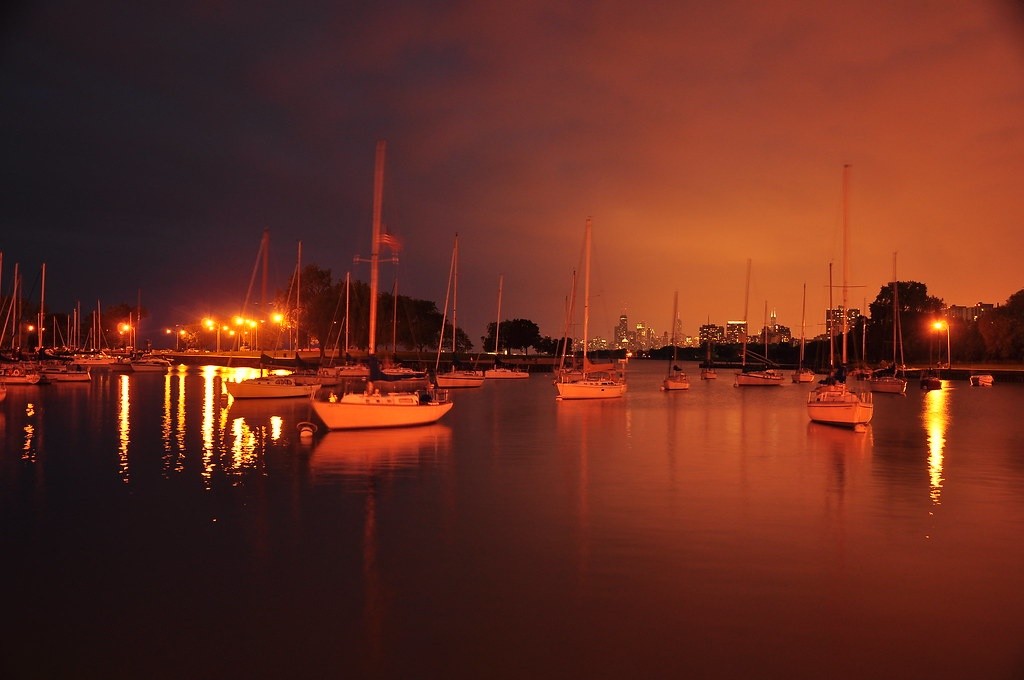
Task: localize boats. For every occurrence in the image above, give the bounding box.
[920,375,941,391]
[970,374,995,387]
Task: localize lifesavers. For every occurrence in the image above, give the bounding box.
[14,369,20,376]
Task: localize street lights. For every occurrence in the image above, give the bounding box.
[165,313,292,351]
[931,320,951,366]
[122,324,135,350]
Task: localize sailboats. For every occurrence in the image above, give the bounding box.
[1,262,176,385]
[791,284,817,383]
[662,292,691,391]
[222,144,530,432]
[554,214,628,401]
[735,257,784,387]
[700,316,719,380]
[806,161,908,429]
